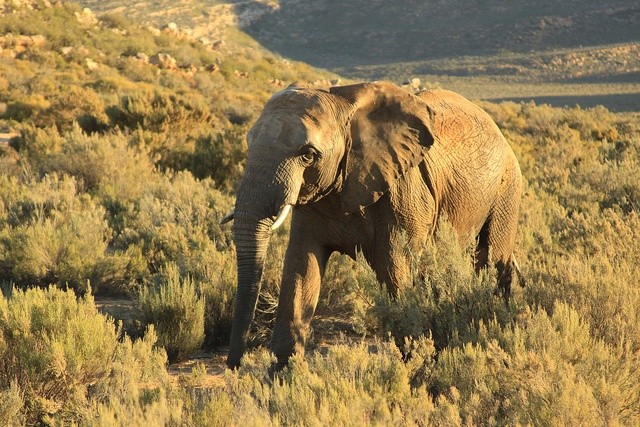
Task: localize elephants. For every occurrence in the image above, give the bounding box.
[226,82,525,383]
